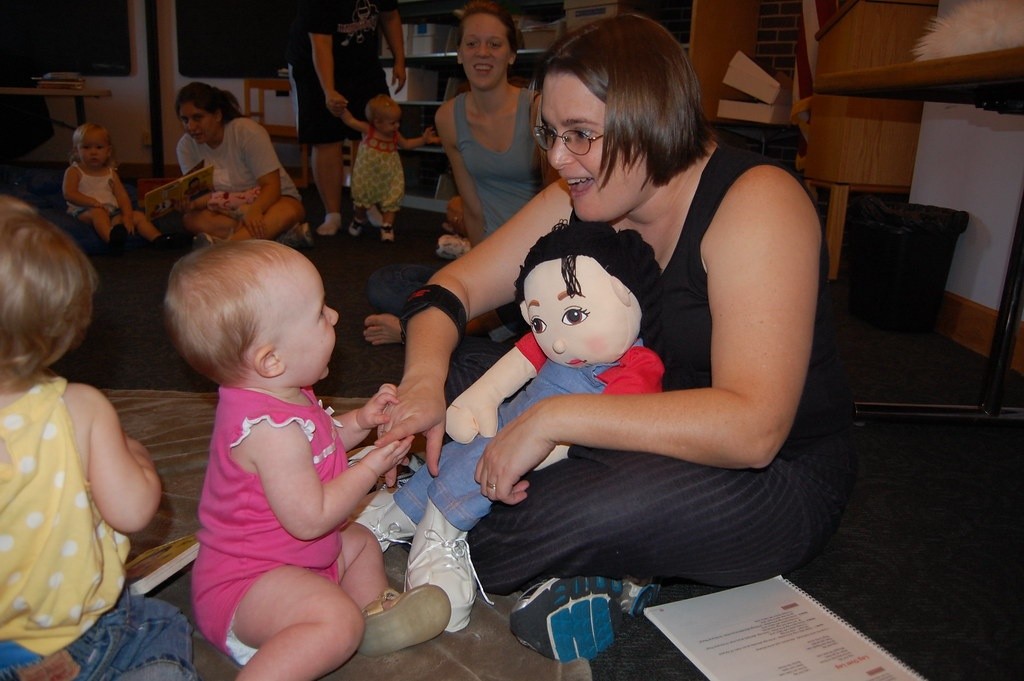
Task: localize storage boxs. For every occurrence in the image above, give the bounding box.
[717,50,793,124]
[377,0,626,103]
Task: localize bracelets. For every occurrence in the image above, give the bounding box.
[400,284,467,352]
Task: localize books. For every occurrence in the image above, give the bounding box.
[123,532,201,597]
[136,159,215,222]
[642,574,927,681]
[37,71,86,90]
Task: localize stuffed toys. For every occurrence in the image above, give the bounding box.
[354,220,669,634]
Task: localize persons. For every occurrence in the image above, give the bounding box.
[63,122,177,249]
[164,238,451,681]
[285,0,406,235]
[174,82,314,250]
[435,0,561,248]
[334,95,441,243]
[0,195,195,681]
[374,12,829,661]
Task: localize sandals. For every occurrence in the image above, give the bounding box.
[357,583,451,655]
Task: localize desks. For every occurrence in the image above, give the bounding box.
[0,86,111,171]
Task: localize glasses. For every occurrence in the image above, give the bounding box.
[532,123,605,156]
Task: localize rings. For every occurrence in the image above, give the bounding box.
[487,481,497,490]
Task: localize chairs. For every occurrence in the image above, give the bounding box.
[242,77,312,187]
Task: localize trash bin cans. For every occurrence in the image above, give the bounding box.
[839,199,967,338]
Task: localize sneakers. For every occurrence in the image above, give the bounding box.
[191,228,234,252]
[274,222,314,250]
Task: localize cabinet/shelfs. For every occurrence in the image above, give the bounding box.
[352,0,763,215]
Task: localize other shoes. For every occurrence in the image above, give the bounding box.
[508,574,663,661]
[380,223,396,242]
[107,224,128,259]
[151,230,193,251]
[349,218,365,236]
[435,233,470,260]
[347,441,425,494]
